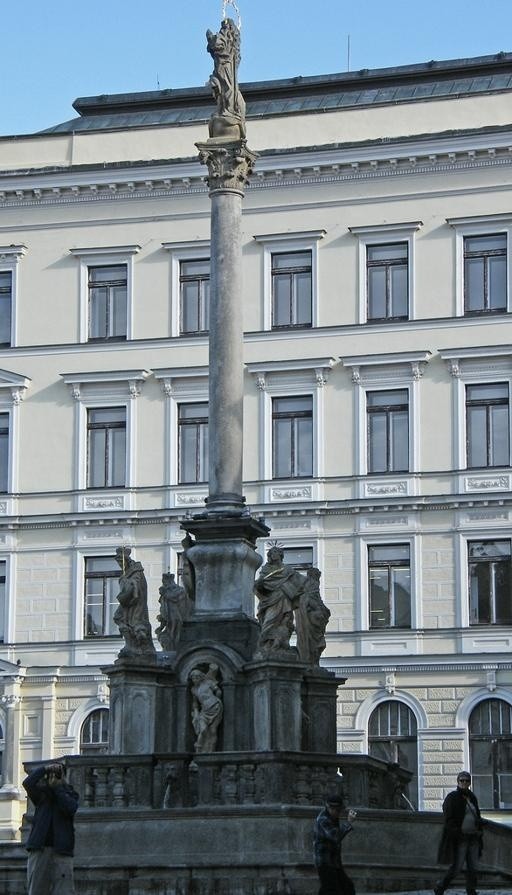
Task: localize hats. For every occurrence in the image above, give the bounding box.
[326,796,346,810]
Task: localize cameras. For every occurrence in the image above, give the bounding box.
[475,818,488,826]
[55,763,66,778]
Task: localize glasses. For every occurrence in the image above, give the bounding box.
[459,779,469,782]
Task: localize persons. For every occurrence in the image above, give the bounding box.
[20,763,82,894]
[154,573,189,656]
[293,566,332,660]
[189,662,225,772]
[432,772,485,895]
[309,788,357,894]
[253,547,306,649]
[110,544,157,661]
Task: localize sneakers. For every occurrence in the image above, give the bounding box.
[434,881,444,895]
